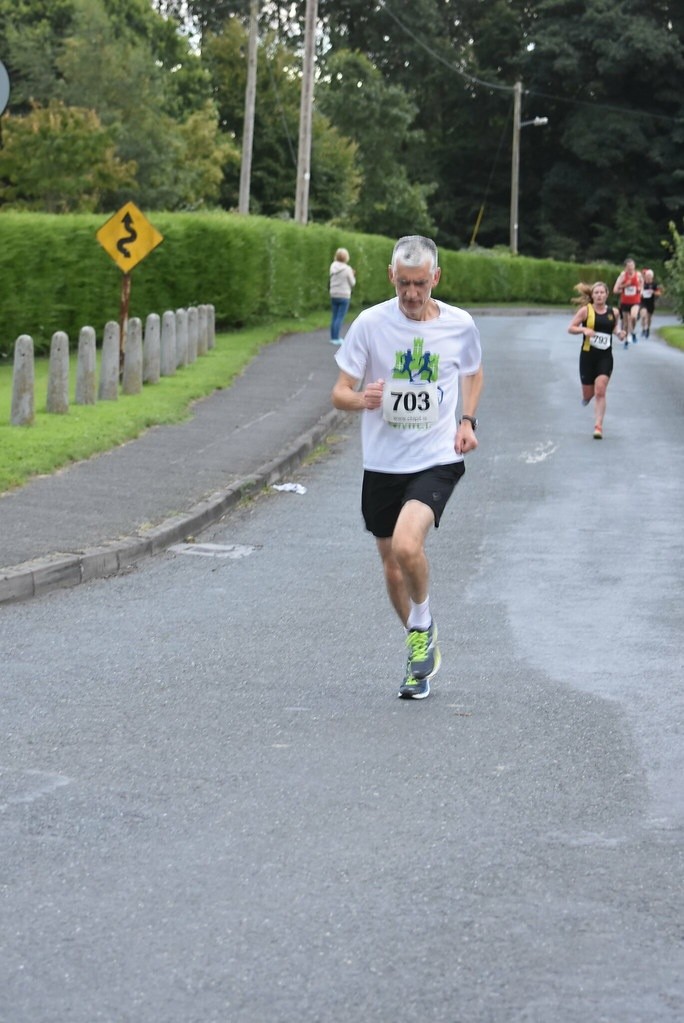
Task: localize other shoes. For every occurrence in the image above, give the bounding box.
[623,340,629,349]
[632,333,637,343]
[642,327,650,339]
[329,337,344,345]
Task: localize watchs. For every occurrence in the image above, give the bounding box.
[459,415,480,431]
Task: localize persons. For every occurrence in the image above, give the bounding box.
[329,235,485,698]
[329,248,358,345]
[612,258,661,350]
[567,281,627,440]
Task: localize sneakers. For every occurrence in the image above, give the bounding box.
[397,650,432,701]
[403,610,440,679]
[592,426,603,439]
[581,396,590,406]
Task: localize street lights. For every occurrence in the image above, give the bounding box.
[510,82,549,259]
